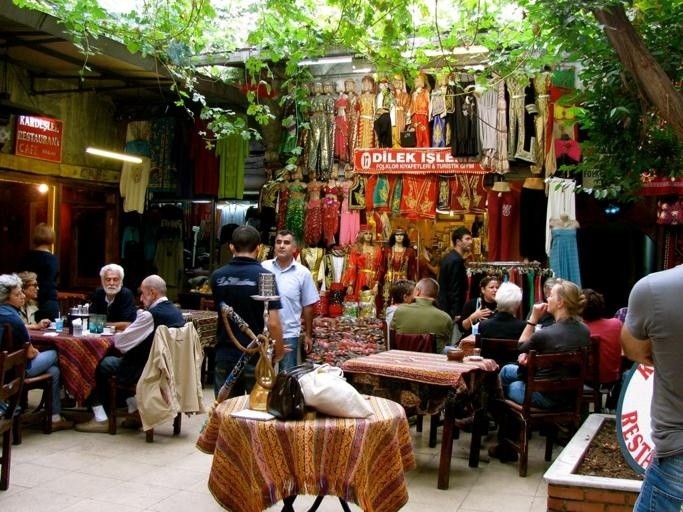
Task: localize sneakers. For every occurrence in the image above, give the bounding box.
[455,416,488,436]
[121,410,142,429]
[52,418,72,431]
[76,419,109,432]
[488,446,519,463]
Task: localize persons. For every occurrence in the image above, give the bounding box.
[85,262,137,331]
[97,274,185,429]
[0,273,76,432]
[209,224,292,403]
[19,270,52,331]
[261,223,321,378]
[14,222,59,322]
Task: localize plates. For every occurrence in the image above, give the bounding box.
[48,327,56,329]
[469,356,483,361]
[182,313,192,316]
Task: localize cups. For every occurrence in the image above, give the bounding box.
[446,349,464,362]
[68,302,114,338]
[50,322,55,328]
[55,318,64,333]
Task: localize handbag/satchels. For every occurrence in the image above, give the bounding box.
[519,353,557,385]
[295,363,374,418]
[267,371,305,420]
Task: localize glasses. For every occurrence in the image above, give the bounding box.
[28,282,37,288]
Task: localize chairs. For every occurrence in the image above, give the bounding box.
[0,339,32,493]
[0,322,53,444]
[472,333,521,369]
[196,296,215,311]
[53,287,87,319]
[105,321,198,444]
[388,327,461,448]
[490,347,592,478]
[583,334,610,414]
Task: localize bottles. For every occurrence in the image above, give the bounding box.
[473,348,480,361]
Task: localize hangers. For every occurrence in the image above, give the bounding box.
[461,259,545,275]
